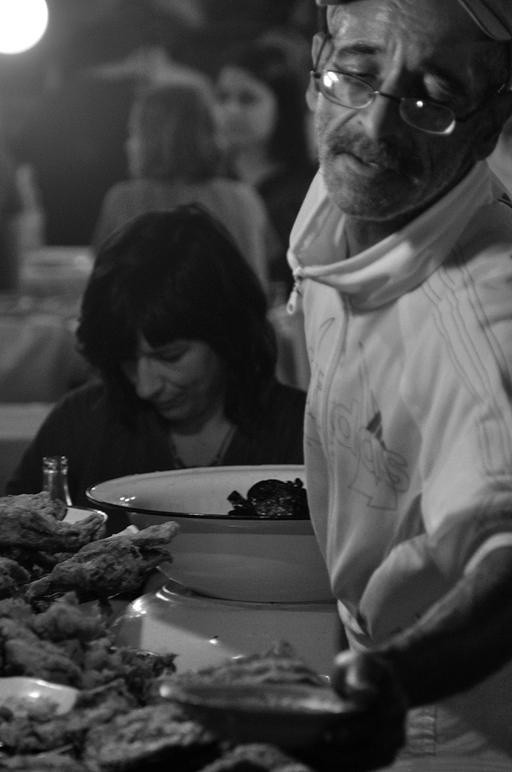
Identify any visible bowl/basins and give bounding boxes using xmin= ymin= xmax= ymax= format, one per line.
xmin=109 ymin=580 xmax=350 ymax=683
xmin=82 ymin=461 xmax=337 ymax=606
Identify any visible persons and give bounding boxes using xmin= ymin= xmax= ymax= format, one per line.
xmin=95 ymin=82 xmax=272 ymax=308
xmin=1 ymin=201 xmax=309 ymax=507
xmin=284 ymin=0 xmax=511 ymax=772
xmin=214 ymin=43 xmax=316 ymax=298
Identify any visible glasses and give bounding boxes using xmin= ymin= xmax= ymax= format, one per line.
xmin=307 ymin=36 xmax=506 ymax=138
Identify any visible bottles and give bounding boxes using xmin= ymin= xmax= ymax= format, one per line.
xmin=40 ymin=455 xmax=75 ymax=508
